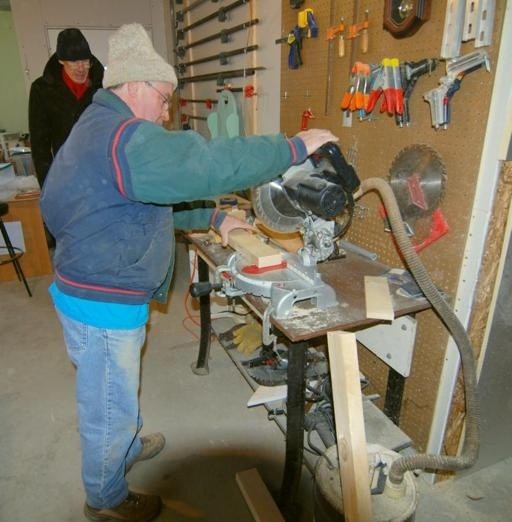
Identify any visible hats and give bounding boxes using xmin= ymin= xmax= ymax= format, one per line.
xmin=102 ymin=22 xmax=178 ymax=89
xmin=57 ymin=28 xmax=93 ymax=61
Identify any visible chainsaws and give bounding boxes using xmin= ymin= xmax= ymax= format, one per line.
xmin=188 ymin=140 xmax=362 ymax=345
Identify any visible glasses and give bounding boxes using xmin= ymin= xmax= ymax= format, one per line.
xmin=142 ymin=80 xmax=174 ymax=110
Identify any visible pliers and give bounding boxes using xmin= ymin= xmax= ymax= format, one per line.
xmin=340 ymin=57 xmax=404 ymax=114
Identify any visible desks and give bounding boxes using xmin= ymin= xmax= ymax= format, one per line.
xmin=179 ymin=208 xmax=451 ymax=521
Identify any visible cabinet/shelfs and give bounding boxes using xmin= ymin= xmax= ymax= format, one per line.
xmin=0 ymin=183 xmax=59 ymax=284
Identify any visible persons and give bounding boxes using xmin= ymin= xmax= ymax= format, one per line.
xmin=38 ymin=21 xmax=342 ymax=522
xmin=27 ymin=28 xmax=105 ymax=192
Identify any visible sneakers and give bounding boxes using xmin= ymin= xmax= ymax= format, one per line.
xmin=84 ymin=491 xmax=163 ymax=522
xmin=125 ymin=432 xmax=166 ymax=473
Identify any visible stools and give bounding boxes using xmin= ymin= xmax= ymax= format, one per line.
xmin=0 ymin=201 xmax=34 ymax=300
